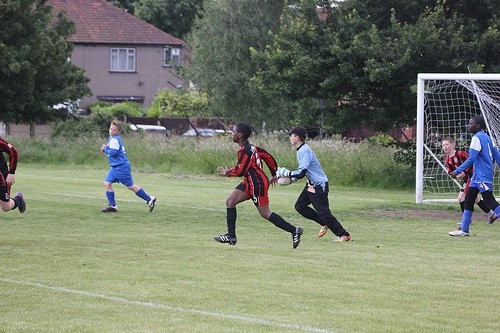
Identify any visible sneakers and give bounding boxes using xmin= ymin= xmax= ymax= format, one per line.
xmin=101 ymin=205 xmax=119 ymax=212
xmin=147 ymin=196 xmax=157 ymax=213
xmin=293 ymin=227 xmax=304 ymax=248
xmin=214 ymin=234 xmax=237 ymax=246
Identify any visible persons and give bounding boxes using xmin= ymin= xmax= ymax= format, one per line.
xmin=441 ymin=137 xmax=495 ymax=225
xmin=276 ymin=126 xmax=353 ymax=242
xmin=214 ymin=123 xmax=303 ymax=249
xmin=449 ymin=116 xmax=500 ymax=237
xmin=0 ymin=136 xmax=26 ymax=213
xmin=100 ymin=120 xmax=157 ymax=213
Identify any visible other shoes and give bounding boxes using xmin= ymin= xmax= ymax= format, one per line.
xmin=449 ymin=230 xmax=469 ymax=236
xmin=317 ymin=224 xmax=329 ymax=237
xmin=487 ymin=212 xmax=498 ymax=224
xmin=333 ymin=235 xmax=350 ymax=242
xmin=16 ymin=192 xmax=26 ymax=213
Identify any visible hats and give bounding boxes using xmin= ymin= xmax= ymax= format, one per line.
xmin=284 ymin=126 xmax=306 ymax=137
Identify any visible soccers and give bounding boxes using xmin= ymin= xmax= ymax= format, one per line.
xmin=278 ymin=177 xmax=291 ymax=186
